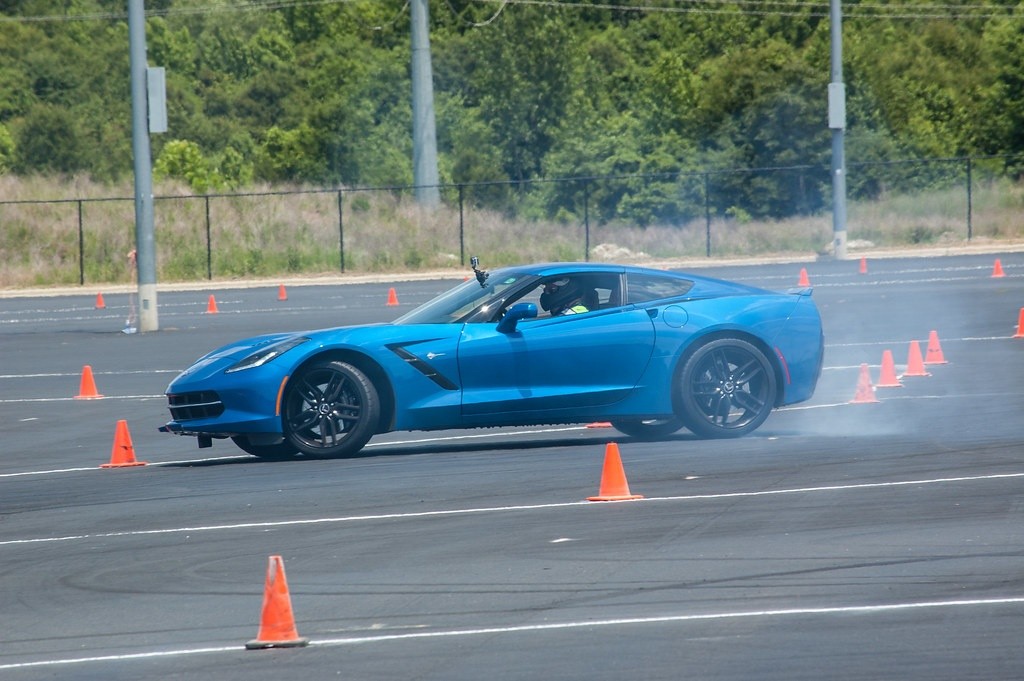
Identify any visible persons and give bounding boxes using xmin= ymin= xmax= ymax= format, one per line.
xmin=541 ymin=277 xmax=593 ymax=316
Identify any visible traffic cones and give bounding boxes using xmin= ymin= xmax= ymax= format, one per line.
xmin=796 ymin=267 xmax=811 ymax=289
xmin=990 ymin=259 xmax=1007 ymax=278
xmin=384 ymin=288 xmax=399 ymax=307
xmin=923 ymin=329 xmax=949 ymax=366
xmin=874 ymin=350 xmax=904 ymax=388
xmin=900 ymin=341 xmax=930 ymax=378
xmin=275 ymin=284 xmax=288 ymax=300
xmin=585 ymin=443 xmax=645 ymax=503
xmin=98 ymin=419 xmax=147 ymax=468
xmin=847 ymin=363 xmax=879 ymax=403
xmin=72 ymin=365 xmax=105 ymax=398
xmin=206 ymin=295 xmax=220 ymax=314
xmin=859 ymin=256 xmax=869 ymax=274
xmin=94 ymin=294 xmax=108 ymax=312
xmin=1010 ymin=307 xmax=1024 ymax=339
xmin=243 ymin=555 xmax=312 ymax=647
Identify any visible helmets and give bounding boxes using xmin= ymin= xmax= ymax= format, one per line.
xmin=540 ymin=277 xmax=578 ymax=311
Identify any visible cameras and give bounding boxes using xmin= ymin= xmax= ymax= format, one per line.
xmin=471 ymin=257 xmax=479 ymax=269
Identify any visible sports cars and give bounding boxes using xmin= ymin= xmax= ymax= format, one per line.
xmin=162 ymin=261 xmax=829 ymax=459
